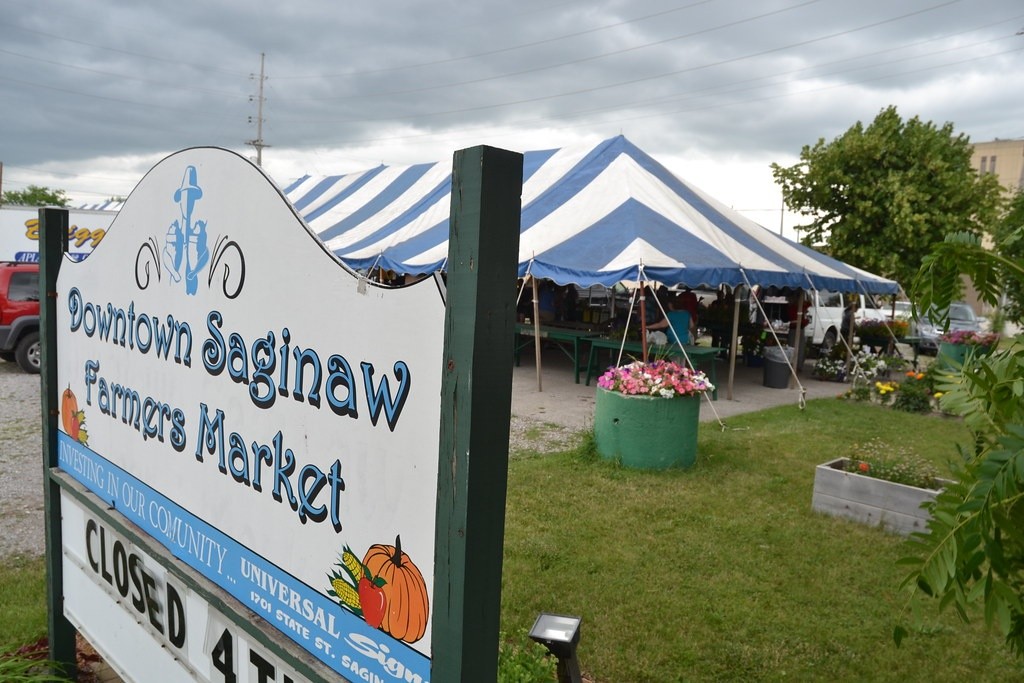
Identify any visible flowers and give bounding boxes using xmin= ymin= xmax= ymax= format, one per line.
xmin=812 ymin=357 xmax=848 ymax=376
xmin=941 ymin=329 xmax=999 ymax=345
xmin=837 ymin=438 xmax=936 ymax=490
xmin=855 ymin=319 xmax=906 ymax=335
xmin=875 ymin=351 xmax=909 ymax=371
xmin=594 ymin=340 xmax=717 ymax=401
xmin=860 ymin=368 xmax=955 ymax=400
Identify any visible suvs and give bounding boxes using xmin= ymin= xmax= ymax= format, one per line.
xmin=0 ymin=259 xmax=42 ymax=374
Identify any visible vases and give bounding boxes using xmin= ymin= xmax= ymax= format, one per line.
xmin=594 ymin=376 xmax=703 ymax=471
xmin=935 ymin=340 xmax=971 ymax=371
xmin=869 ymin=387 xmax=963 ymax=418
xmin=814 ymin=369 xmax=845 ymax=384
xmin=869 ymin=365 xmax=907 ymax=381
xmin=860 ymin=332 xmax=905 ymax=339
xmin=811 ymin=458 xmax=959 ymax=544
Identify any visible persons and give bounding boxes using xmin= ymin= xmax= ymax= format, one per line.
xmin=513 ymin=275 xmax=766 ymax=366
xmin=833 ymin=299 xmax=861 ymax=358
xmin=786 ymin=288 xmax=815 ymax=370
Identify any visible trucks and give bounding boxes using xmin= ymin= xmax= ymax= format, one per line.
xmin=1 ymin=205 xmax=120 ymax=265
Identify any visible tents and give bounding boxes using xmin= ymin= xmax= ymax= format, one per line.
xmin=261 ymin=130 xmax=906 ymax=397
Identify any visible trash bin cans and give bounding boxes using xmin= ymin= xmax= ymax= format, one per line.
xmin=762 ymin=344 xmax=796 ymax=389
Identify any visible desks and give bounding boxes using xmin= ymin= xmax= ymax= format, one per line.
xmin=515 ymin=323 xmax=604 ymax=384
xmin=580 ymin=337 xmax=726 ymax=401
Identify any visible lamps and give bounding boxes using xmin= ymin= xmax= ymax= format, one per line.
xmin=527 ymin=613 xmax=584 ymax=683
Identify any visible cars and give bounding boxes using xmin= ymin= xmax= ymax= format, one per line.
xmin=738 ymin=291 xmax=987 ymax=353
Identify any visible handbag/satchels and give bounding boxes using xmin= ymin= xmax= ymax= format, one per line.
xmin=695 ymin=335 xmax=712 ymax=348
